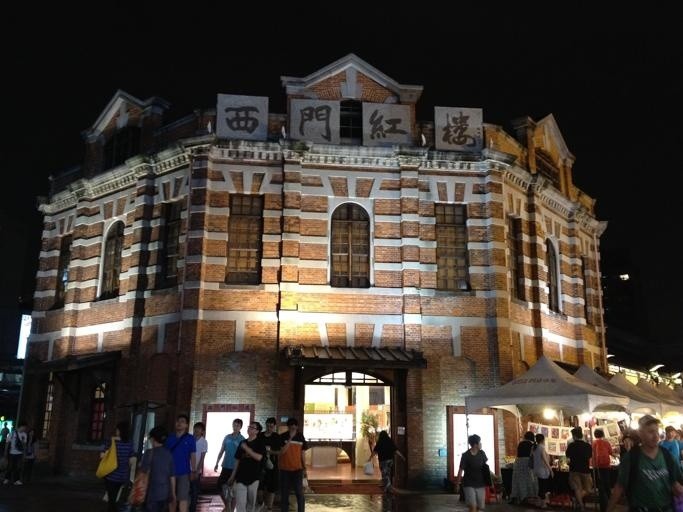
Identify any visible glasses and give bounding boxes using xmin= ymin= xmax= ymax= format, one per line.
xmin=248 ymin=426 xmax=257 ymax=430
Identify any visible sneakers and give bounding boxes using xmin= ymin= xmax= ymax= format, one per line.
xmin=13 ymin=479 xmax=24 ymax=486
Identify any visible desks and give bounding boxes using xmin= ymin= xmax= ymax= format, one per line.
xmin=501 ymin=466 xmax=618 ymax=511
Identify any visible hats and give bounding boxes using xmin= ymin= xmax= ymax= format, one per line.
xmin=639 ymin=415 xmax=660 ymax=425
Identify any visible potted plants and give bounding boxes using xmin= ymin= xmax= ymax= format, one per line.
xmin=70 ymin=436 xmax=104 ymax=472
xmin=360 ymin=409 xmax=380 ymax=468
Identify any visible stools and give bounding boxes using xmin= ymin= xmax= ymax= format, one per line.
xmin=486 ymin=480 xmax=498 ymax=505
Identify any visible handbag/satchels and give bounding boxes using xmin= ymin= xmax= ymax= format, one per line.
xmin=262 ymin=450 xmax=282 ymax=484
xmin=528 ymin=444 xmax=537 ymax=469
xmin=96 ymin=437 xmax=118 ymax=479
xmin=363 ymin=460 xmax=374 ymax=475
xmin=14 ymin=428 xmax=25 ymax=451
xmin=128 ymin=449 xmax=153 ymax=506
xmin=480 ymin=449 xmax=492 ymax=487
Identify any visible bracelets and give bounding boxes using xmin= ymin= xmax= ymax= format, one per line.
xmin=303 ymin=466 xmax=306 ymax=469
xmin=398 ymin=453 xmax=402 ymax=458
xmin=191 ymin=469 xmax=195 ymax=473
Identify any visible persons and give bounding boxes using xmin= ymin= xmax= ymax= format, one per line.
xmin=565 ymin=426 xmax=594 ymax=511
xmin=590 ymin=428 xmax=612 ymax=512
xmin=214 ymin=419 xmax=245 ymax=512
xmin=529 ymin=433 xmax=555 ymax=511
xmin=456 ymin=434 xmax=489 ymax=511
xmin=366 ymin=429 xmax=405 ymax=493
xmin=95 ymin=421 xmax=136 ymax=512
xmin=225 ymin=421 xmax=266 ymax=512
xmin=134 ymin=425 xmax=177 ymax=511
xmin=507 ymin=430 xmax=536 ymax=505
xmin=188 ymin=421 xmax=207 ymax=512
xmin=165 ymin=414 xmax=197 ymax=512
xmin=257 ymin=416 xmax=279 ymax=512
xmin=268 ymin=417 xmax=307 ymax=512
xmin=0 ymin=422 xmax=39 ymax=487
xmin=605 ymin=414 xmax=682 ymax=512
